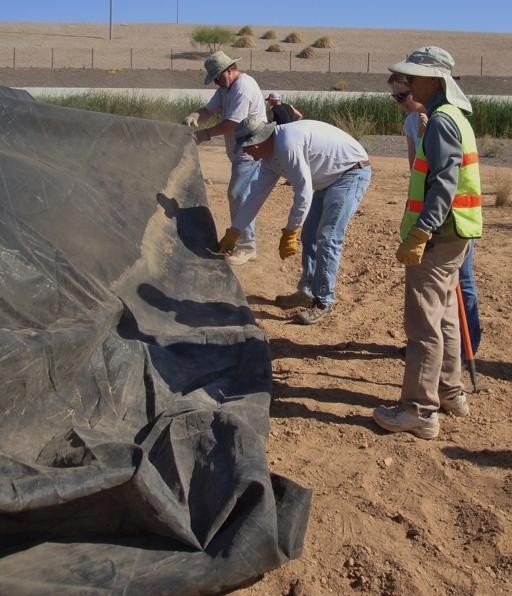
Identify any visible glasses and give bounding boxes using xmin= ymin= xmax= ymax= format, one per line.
xmin=391 ymin=90 xmax=410 ymax=101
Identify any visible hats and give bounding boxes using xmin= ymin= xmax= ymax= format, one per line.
xmin=265 ymin=91 xmax=281 ymax=101
xmin=203 ymin=51 xmax=243 ymax=85
xmin=388 ymin=46 xmax=472 ymax=116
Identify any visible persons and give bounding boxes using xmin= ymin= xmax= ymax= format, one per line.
xmin=374 ymin=46 xmax=484 ymax=439
xmin=182 ymin=51 xmax=269 ymax=264
xmin=387 ymin=72 xmax=480 ymax=373
xmin=214 ymin=116 xmax=373 ymax=325
xmin=266 ymin=92 xmax=302 ymax=124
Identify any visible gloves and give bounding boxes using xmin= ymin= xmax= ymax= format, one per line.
xmin=192 ymin=127 xmax=210 ymax=145
xmin=183 ymin=112 xmax=200 ymax=128
xmin=218 ymin=228 xmax=240 ymax=256
xmin=234 ymin=116 xmax=277 ymax=154
xmin=279 ymin=228 xmax=300 ymax=260
xmin=395 ymin=225 xmax=433 ymax=265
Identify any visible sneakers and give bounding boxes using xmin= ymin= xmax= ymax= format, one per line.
xmin=296 ymin=303 xmax=333 ymax=325
xmin=275 ymin=290 xmax=312 ymax=308
xmin=224 ymin=247 xmax=256 ymax=265
xmin=373 ymin=392 xmax=470 ymax=439
